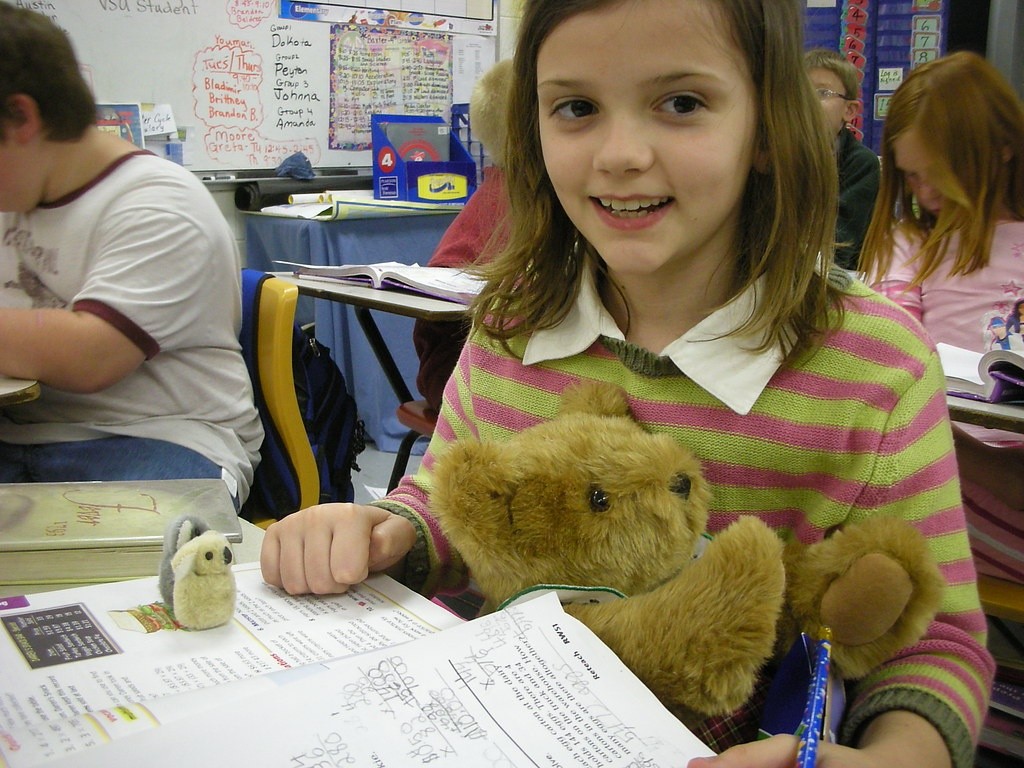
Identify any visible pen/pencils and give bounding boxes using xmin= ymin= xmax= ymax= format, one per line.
xmin=794 ymin=625 xmax=834 ymax=767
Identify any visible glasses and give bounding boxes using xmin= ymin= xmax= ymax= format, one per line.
xmin=815 ymin=88 xmax=855 ymax=101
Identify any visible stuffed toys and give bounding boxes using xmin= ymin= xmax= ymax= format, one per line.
xmin=428 ymin=379 xmax=946 ymax=754
xmin=159 ymin=514 xmax=236 ymax=629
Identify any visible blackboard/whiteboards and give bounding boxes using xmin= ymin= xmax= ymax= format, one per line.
xmin=0 ymin=0 xmax=501 ymax=193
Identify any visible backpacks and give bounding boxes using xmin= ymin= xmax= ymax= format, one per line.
xmin=237 ymin=265 xmax=368 ymax=517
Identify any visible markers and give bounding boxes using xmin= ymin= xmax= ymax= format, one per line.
xmin=201 ymin=174 xmax=237 ymax=179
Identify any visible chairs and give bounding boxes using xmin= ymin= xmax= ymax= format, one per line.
xmin=236 ymin=264 xmax=324 ymax=535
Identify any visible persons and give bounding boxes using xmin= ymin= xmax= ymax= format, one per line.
xmin=803 ymin=49 xmax=880 ymax=270
xmin=413 ymin=58 xmax=513 ymax=421
xmin=0 ymin=0 xmax=264 ymax=513
xmin=259 ymin=1 xmax=997 ymax=768
xmin=857 ymin=51 xmax=1024 ymax=586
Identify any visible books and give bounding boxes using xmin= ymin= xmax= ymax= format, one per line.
xmin=978 ymin=674 xmax=1024 ymax=759
xmin=936 ymin=341 xmax=1024 ymax=404
xmin=272 ymin=260 xmax=489 ymax=305
xmin=0 ymin=479 xmax=244 ymax=586
xmin=0 ymin=560 xmax=717 ymax=768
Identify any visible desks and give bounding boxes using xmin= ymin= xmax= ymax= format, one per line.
xmin=268 ymin=266 xmax=472 ymax=497
xmin=243 ymin=204 xmax=467 ymax=458
